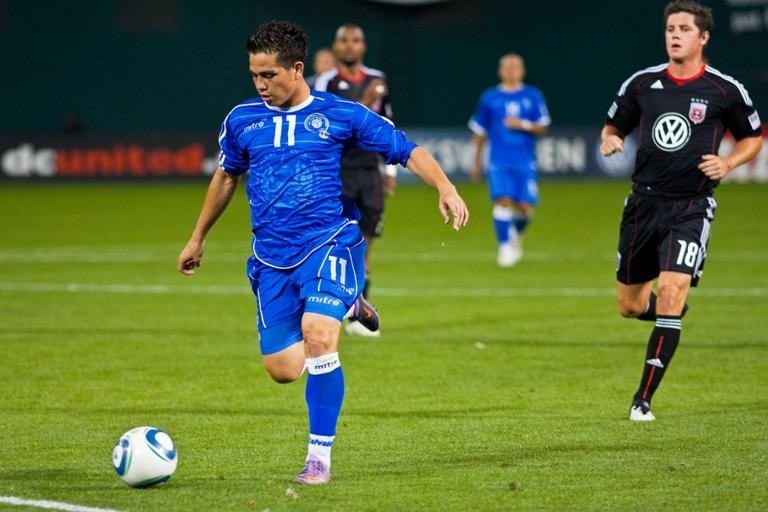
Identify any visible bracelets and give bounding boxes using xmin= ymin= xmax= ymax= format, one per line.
xmin=521 ymin=119 xmax=532 ymax=131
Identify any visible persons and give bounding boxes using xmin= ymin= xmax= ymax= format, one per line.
xmin=305 ymin=47 xmax=336 ymax=91
xmin=312 ymin=24 xmax=390 ymax=335
xmin=177 ymin=20 xmax=469 ymax=484
xmin=467 ymin=53 xmax=552 ymax=268
xmin=384 ymin=95 xmax=396 ymax=195
xmin=600 ymin=1 xmax=763 ymax=423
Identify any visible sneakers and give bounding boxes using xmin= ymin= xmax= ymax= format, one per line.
xmin=292 ymin=454 xmax=330 ymax=487
xmin=497 ymin=243 xmax=514 ymax=268
xmin=509 ymin=226 xmax=521 ymax=262
xmin=345 ymin=320 xmax=380 ymax=337
xmin=348 ymin=292 xmax=379 ymax=332
xmin=628 ymin=391 xmax=655 ymax=423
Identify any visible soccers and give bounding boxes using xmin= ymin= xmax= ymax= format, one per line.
xmin=110 ymin=425 xmax=179 ymax=488
